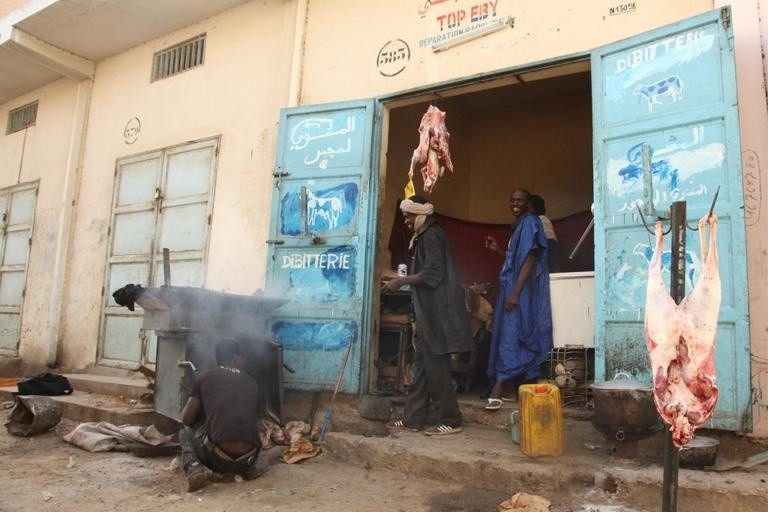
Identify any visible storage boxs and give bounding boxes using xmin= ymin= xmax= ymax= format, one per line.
xmin=548 ymin=270 xmax=596 ymax=349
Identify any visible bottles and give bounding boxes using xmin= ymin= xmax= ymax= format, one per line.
xmin=397 ymin=265 xmax=408 ymax=291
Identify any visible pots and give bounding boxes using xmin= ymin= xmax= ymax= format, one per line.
xmin=587 ymin=373 xmax=659 ymax=434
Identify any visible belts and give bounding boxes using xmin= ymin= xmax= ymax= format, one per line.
xmin=203 ymin=436 xmax=257 ymax=462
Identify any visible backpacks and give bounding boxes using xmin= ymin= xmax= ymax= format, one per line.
xmin=13 ymin=372 xmax=73 ymax=395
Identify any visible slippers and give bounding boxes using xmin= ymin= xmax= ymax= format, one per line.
xmin=501 ymin=397 xmax=518 ymax=401
xmin=485 ymin=398 xmax=504 ymax=410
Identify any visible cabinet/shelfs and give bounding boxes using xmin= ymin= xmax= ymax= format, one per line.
xmin=375 ymin=286 xmax=480 ymax=395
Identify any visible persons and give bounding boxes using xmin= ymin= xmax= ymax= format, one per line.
xmin=174 ymin=338 xmax=266 ymax=491
xmin=484 ymin=187 xmax=555 ymax=411
xmin=528 ymin=193 xmax=566 ymax=274
xmin=378 ymin=196 xmax=476 ymax=434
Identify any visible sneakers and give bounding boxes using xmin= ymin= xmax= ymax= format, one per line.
xmin=386 ymin=415 xmax=419 ymax=432
xmin=187 ymin=465 xmax=213 ymax=488
xmin=423 ymin=424 xmax=462 ymax=435
xmin=245 ymin=463 xmax=264 ymax=480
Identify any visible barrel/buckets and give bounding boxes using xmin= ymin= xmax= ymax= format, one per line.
xmin=518 ymin=384 xmax=564 ymax=458
